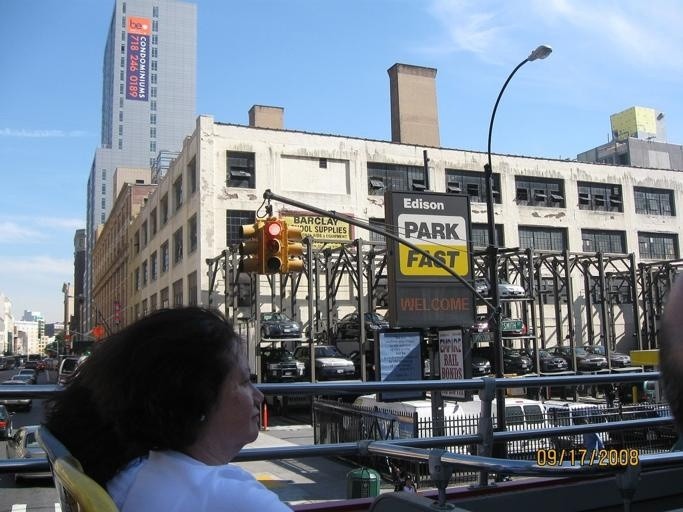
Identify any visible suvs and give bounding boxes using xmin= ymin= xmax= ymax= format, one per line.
xmin=293 ymin=344 xmax=355 ymax=380
xmin=0 ymin=379 xmax=33 ymax=412
xmin=540 ymin=397 xmax=612 ymax=445
xmin=260 ymin=346 xmax=305 ymax=382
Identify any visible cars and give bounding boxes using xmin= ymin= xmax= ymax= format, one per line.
xmin=610 ymin=404 xmax=676 ymax=444
xmin=4 ymin=423 xmax=47 ymax=461
xmin=0 ymin=404 xmax=17 ymax=439
xmin=349 ymin=346 xmax=374 ymax=378
xmin=0 ymin=352 xmax=77 ymax=386
xmin=259 ymin=311 xmax=303 ymax=339
xmin=473 ymin=277 xmax=631 ymax=375
xmin=334 ymin=310 xmax=390 ymax=339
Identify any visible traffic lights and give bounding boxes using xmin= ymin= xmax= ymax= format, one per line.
xmin=284 ymin=221 xmax=305 ymax=274
xmin=260 ymin=220 xmax=289 ymax=275
xmin=238 ymin=223 xmax=264 ymax=275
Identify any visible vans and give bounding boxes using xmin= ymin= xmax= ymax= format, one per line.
xmin=490 ymin=397 xmax=552 ymax=454
xmin=641 ymin=380 xmax=656 ymax=401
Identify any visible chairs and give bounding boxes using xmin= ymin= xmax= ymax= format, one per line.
xmin=34 ymin=426 xmax=121 ymax=512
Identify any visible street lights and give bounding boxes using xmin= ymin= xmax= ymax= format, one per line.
xmin=483 ymin=43 xmax=553 ymax=483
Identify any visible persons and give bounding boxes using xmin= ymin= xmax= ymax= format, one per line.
xmin=401 ymin=478 xmax=416 ymax=493
xmin=393 ymin=472 xmax=415 ymax=492
xmin=38 ymin=303 xmax=294 ymax=512
xmin=657 ymin=267 xmax=682 ymax=436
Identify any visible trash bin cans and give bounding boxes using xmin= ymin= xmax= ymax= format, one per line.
xmin=346 ymin=467 xmax=380 ymax=499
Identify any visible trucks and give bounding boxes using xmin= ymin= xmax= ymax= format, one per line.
xmin=339 ymin=393 xmax=470 ymax=469
